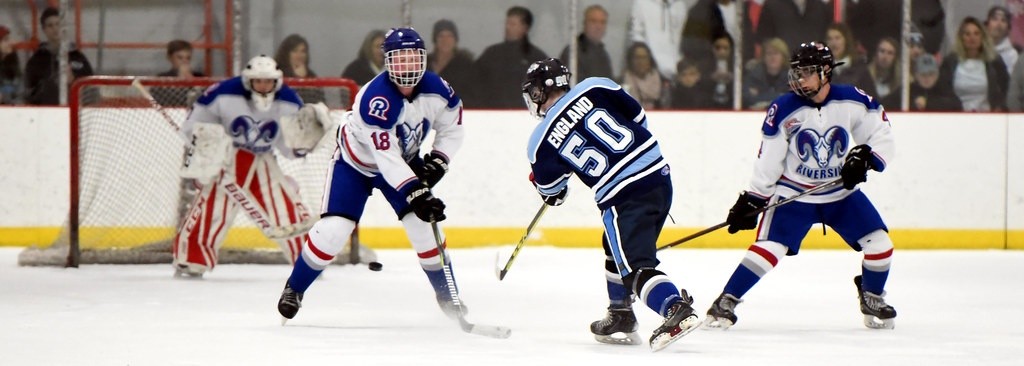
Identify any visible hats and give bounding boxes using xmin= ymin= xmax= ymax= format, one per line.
xmin=916 ymin=54 xmax=937 ymax=72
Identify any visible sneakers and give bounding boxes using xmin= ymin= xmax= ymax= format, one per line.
xmin=855 ymin=274 xmax=896 ymax=329
xmin=708 ymin=292 xmax=743 ymax=330
xmin=440 ymin=298 xmax=468 ymax=323
xmin=277 ymin=276 xmax=304 ymax=328
xmin=649 ymin=288 xmax=702 ymax=353
xmin=590 ymin=293 xmax=640 ymax=345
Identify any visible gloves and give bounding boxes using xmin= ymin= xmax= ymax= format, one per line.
xmin=840 ymin=143 xmax=872 ymax=190
xmin=407 ymin=185 xmax=445 ymax=223
xmin=542 ymin=187 xmax=568 ymax=206
xmin=727 ymin=189 xmax=771 ymax=235
xmin=417 ymin=154 xmax=448 ymax=188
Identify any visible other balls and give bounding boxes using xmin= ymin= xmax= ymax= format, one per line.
xmin=369 ymin=262 xmax=382 ymax=271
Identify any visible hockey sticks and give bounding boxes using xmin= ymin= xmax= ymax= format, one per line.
xmin=499 ymin=203 xmax=547 ymax=281
xmin=429 ymin=213 xmax=511 ymax=340
xmin=131 ymin=79 xmax=329 ymax=242
xmin=656 ymin=178 xmax=844 ymax=252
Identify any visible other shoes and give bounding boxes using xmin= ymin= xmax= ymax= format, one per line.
xmin=176 ymin=263 xmax=203 ymax=280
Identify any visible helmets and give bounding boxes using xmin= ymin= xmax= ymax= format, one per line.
xmin=521 ymin=57 xmax=571 ymax=120
xmin=788 ymin=41 xmax=833 ymax=99
xmin=380 ymin=26 xmax=427 ymax=89
xmin=240 ymin=54 xmax=284 ymax=113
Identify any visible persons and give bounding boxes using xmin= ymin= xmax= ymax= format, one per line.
xmin=468 ymin=6 xmax=551 ymax=110
xmin=340 ymin=30 xmax=387 ymax=109
xmin=426 ymin=19 xmax=470 ymax=110
xmin=701 ymin=41 xmax=897 ymax=332
xmin=277 ymin=28 xmax=467 ymax=326
xmin=665 ymin=58 xmax=715 ymax=111
xmin=617 ymin=42 xmax=675 ymax=111
xmin=0 ymin=25 xmax=22 ymax=106
xmin=149 ymin=40 xmax=210 ymax=108
xmin=560 ymin=5 xmax=618 ymax=85
xmin=172 ymin=54 xmax=332 ymax=278
xmin=25 ymin=7 xmax=99 ymax=106
xmin=908 ymin=6 xmax=1024 ymax=112
xmin=521 ymin=58 xmax=702 ymax=351
xmin=274 ymin=33 xmax=329 ymax=107
xmin=681 ymin=0 xmax=903 ymax=112
